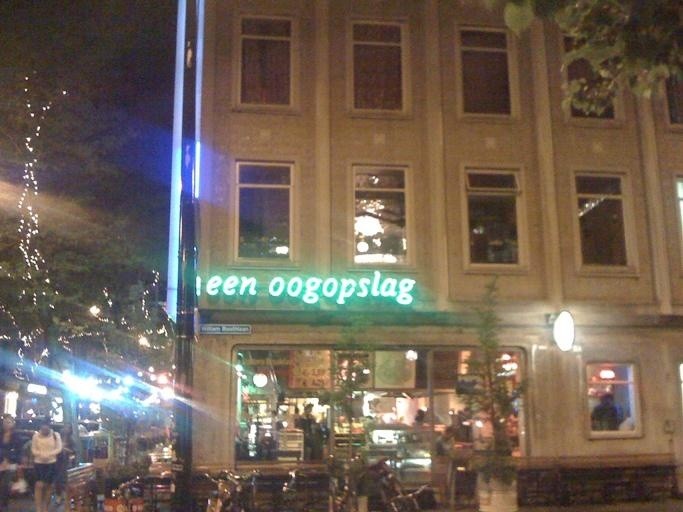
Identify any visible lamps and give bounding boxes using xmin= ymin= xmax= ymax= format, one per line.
xmin=404 ymin=350 xmax=417 ymax=361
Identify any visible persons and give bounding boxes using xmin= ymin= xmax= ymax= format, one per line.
xmin=31 ymin=422 xmax=63 ymax=512
xmin=0 ymin=428 xmax=21 ymax=510
xmin=55 ymin=423 xmax=75 ymax=505
xmin=591 ymin=392 xmax=623 ymax=430
xmin=300 ymin=404 xmax=314 ymax=420
xmin=436 ymin=428 xmax=456 ymax=452
xmin=415 ymin=409 xmax=424 ymax=420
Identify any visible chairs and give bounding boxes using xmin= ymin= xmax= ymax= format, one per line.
xmin=107 ymin=466 xmax=433 ymax=512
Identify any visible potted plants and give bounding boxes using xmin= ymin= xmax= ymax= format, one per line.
xmin=433 ymin=271 xmax=531 ymax=512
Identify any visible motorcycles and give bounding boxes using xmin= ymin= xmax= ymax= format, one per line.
xmin=193 ymin=453 xmax=426 ymax=511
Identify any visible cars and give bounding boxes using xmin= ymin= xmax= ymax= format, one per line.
xmin=6 ymin=417 xmax=98 ymax=454
